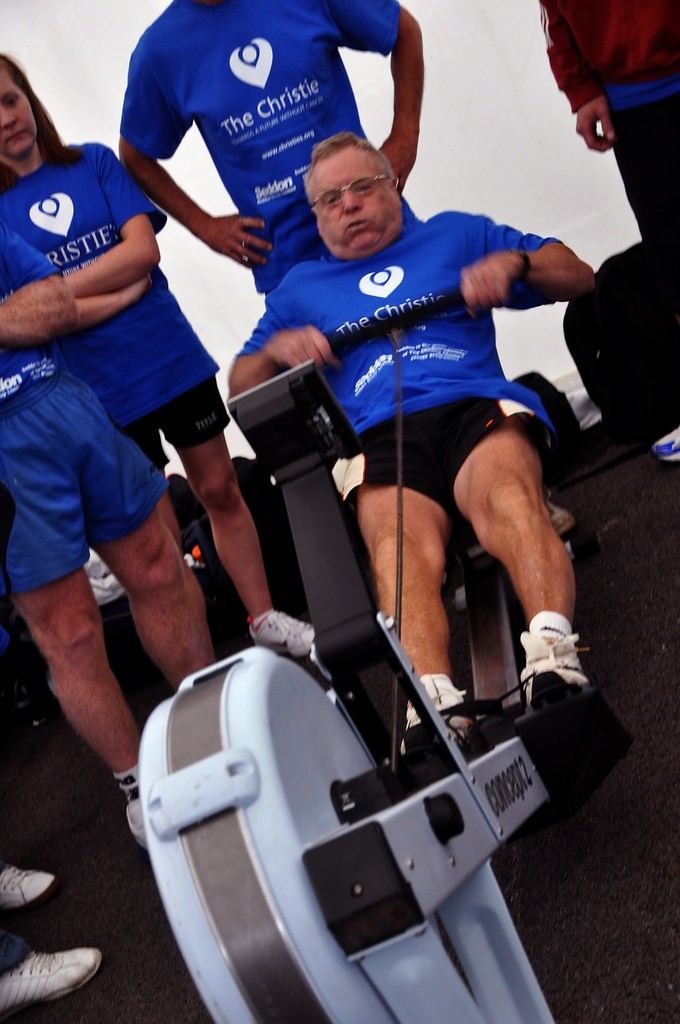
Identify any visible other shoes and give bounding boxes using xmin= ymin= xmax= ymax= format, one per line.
xmin=545 ymin=499 xmax=575 ymax=534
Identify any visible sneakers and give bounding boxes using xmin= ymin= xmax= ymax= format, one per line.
xmin=651 ymin=425 xmax=680 ymax=461
xmin=0 ymin=864 xmax=59 ymax=912
xmin=0 ymin=947 xmax=102 ymax=1021
xmin=519 ymin=631 xmax=589 ymax=710
xmin=399 ymin=673 xmax=472 ymax=756
xmin=247 ymin=610 xmax=315 ymax=656
xmin=126 ymin=805 xmax=148 ymax=851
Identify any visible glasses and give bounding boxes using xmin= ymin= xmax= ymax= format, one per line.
xmin=313 ymin=174 xmax=391 ymax=207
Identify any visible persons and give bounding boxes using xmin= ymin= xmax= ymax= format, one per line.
xmin=540 ymin=0 xmax=680 ymax=460
xmin=0 ymin=54 xmax=317 ymax=846
xmin=228 ymin=135 xmax=593 ymax=748
xmin=117 ymin=0 xmax=576 ymax=609
xmin=0 ymin=864 xmax=102 ymax=1020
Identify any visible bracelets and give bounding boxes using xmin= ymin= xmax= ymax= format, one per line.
xmin=512 ymin=250 xmax=530 ymax=283
